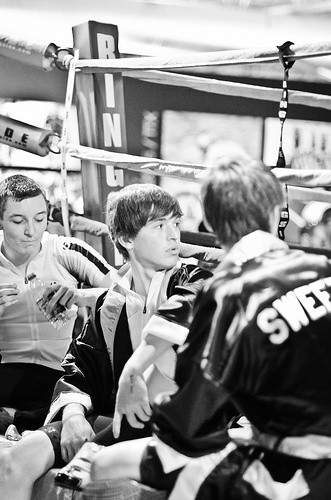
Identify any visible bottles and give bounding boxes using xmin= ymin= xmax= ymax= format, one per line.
xmin=25 ymin=273 xmax=72 ymax=330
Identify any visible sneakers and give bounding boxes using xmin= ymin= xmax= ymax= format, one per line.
xmin=54 ymin=441 xmax=108 ymax=491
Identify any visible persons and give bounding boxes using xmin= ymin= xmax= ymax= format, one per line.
xmin=1 ymin=176 xmax=122 ymax=436
xmin=1 ymin=182 xmax=215 ymax=500
xmin=89 ymin=159 xmax=331 ymax=500
